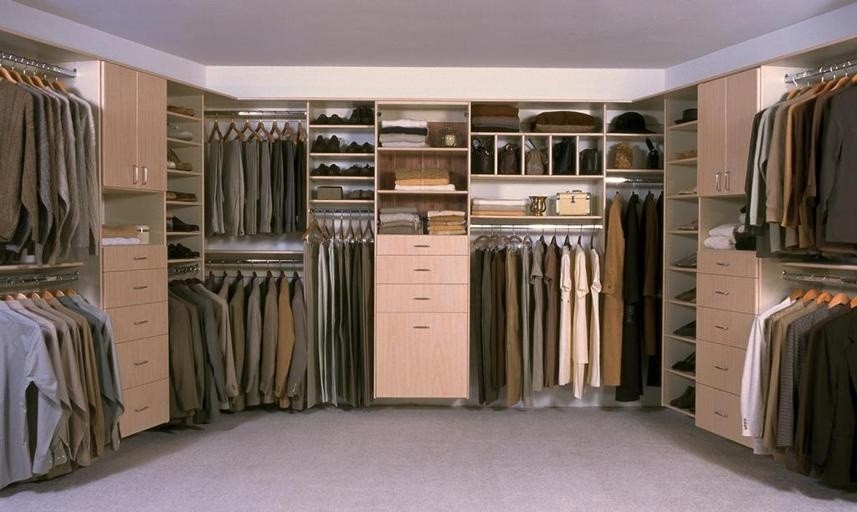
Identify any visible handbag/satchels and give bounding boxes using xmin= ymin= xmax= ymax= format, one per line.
xmin=498 ymin=143 xmax=521 ymax=175
xmin=426 ymin=120 xmax=468 ymax=148
xmin=610 ymin=142 xmax=633 ymax=169
xmin=554 ymin=136 xmax=575 ymax=175
xmin=644 ymin=139 xmax=659 ymax=169
xmin=579 ymin=148 xmax=600 ymax=175
xmin=471 ymin=137 xmax=494 ymax=174
xmin=525 ymin=138 xmax=546 ymax=175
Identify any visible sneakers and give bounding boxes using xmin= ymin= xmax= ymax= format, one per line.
xmin=167 ymin=101 xmax=201 ymax=260
xmin=310 ymin=103 xmax=376 ymax=200
xmin=667 ymin=148 xmax=698 ymax=413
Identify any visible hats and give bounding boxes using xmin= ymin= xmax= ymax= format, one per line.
xmin=614 ymin=112 xmax=655 ymax=134
xmin=674 ymin=109 xmax=697 ymax=124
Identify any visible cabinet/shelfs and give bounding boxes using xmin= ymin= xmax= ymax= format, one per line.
xmin=1 ymin=28 xmax=857 ymax=453
